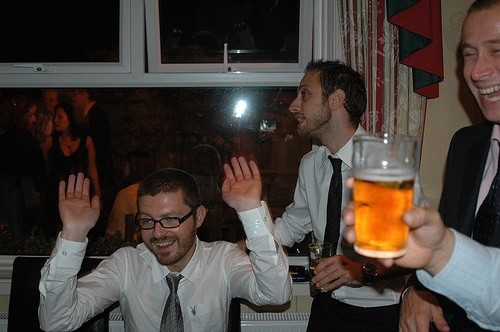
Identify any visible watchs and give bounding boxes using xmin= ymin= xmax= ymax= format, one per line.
xmin=360 ymin=260 xmax=378 ymax=285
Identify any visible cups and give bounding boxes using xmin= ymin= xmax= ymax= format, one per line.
xmin=351 ymin=133 xmax=416 ymax=259
xmin=307 ymin=242 xmax=332 ymax=299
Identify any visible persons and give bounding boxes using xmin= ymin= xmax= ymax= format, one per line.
xmin=11 ymin=88 xmax=286 ymax=249
xmin=398 ymin=0 xmax=500 ymax=332
xmin=341 ymin=176 xmax=500 ymax=331
xmin=233 ymin=59 xmax=430 ymax=332
xmin=38 ymin=157 xmax=293 ymax=332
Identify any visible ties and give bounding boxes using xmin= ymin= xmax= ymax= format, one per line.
xmin=323 ymin=156 xmax=342 ymax=259
xmin=160 ymin=274 xmax=186 ymax=332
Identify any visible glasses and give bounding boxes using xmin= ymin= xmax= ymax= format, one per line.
xmin=133 ymin=208 xmax=195 ymax=230
